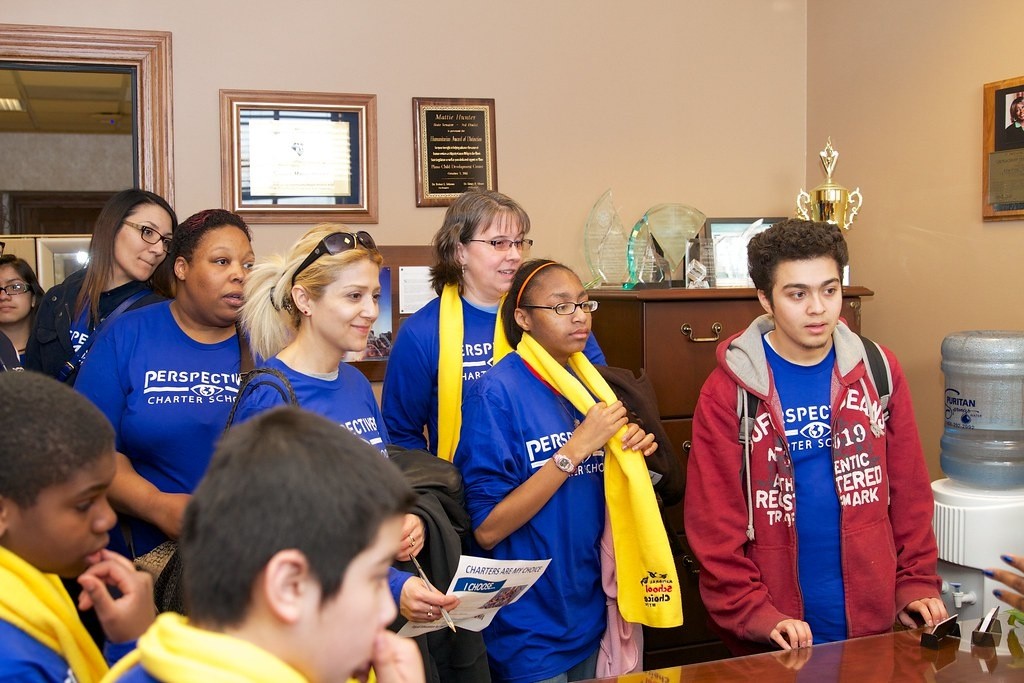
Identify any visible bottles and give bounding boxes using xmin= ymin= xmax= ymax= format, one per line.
xmin=940 ymin=330 xmax=1024 ymax=490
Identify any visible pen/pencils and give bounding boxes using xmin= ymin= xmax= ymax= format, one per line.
xmin=407 ymin=552 xmax=456 ymax=634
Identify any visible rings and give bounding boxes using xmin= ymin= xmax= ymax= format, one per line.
xmin=409 ymin=535 xmax=416 ymax=546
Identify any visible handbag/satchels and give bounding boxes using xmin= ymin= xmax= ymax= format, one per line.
xmin=132 ymin=537 xmax=187 ymax=616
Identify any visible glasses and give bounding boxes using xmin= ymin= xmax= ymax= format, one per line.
xmin=121 ymin=220 xmax=173 ymax=254
xmin=519 ymin=301 xmax=600 ymax=314
xmin=469 ymin=239 xmax=533 ymax=249
xmin=0 ymin=284 xmax=30 ymax=295
xmin=292 ymin=230 xmax=375 ymax=287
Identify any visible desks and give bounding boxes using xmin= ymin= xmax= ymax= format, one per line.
xmin=577 ymin=607 xmax=1024 ymax=683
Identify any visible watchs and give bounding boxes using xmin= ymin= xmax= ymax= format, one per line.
xmin=552 ymin=452 xmax=577 ymax=473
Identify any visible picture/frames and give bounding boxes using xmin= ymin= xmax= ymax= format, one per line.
xmin=705 ymin=216 xmax=789 ymax=287
xmin=981 ymin=76 xmax=1024 ymax=223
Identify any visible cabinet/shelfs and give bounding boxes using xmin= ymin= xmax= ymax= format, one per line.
xmin=585 ymin=285 xmax=875 ymax=672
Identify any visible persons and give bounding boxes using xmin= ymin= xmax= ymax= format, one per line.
xmin=453 ymin=259 xmax=657 ymax=683
xmin=98 ymin=405 xmax=425 ymax=683
xmin=382 ymin=189 xmax=607 ymax=463
xmin=982 ymin=554 xmax=1024 ymax=611
xmin=684 ymin=218 xmax=949 ymax=650
xmin=0 ymin=188 xmax=459 ymax=654
xmin=0 ymin=371 xmax=157 ymax=683
xmin=1005 ymin=97 xmax=1024 ymax=150
